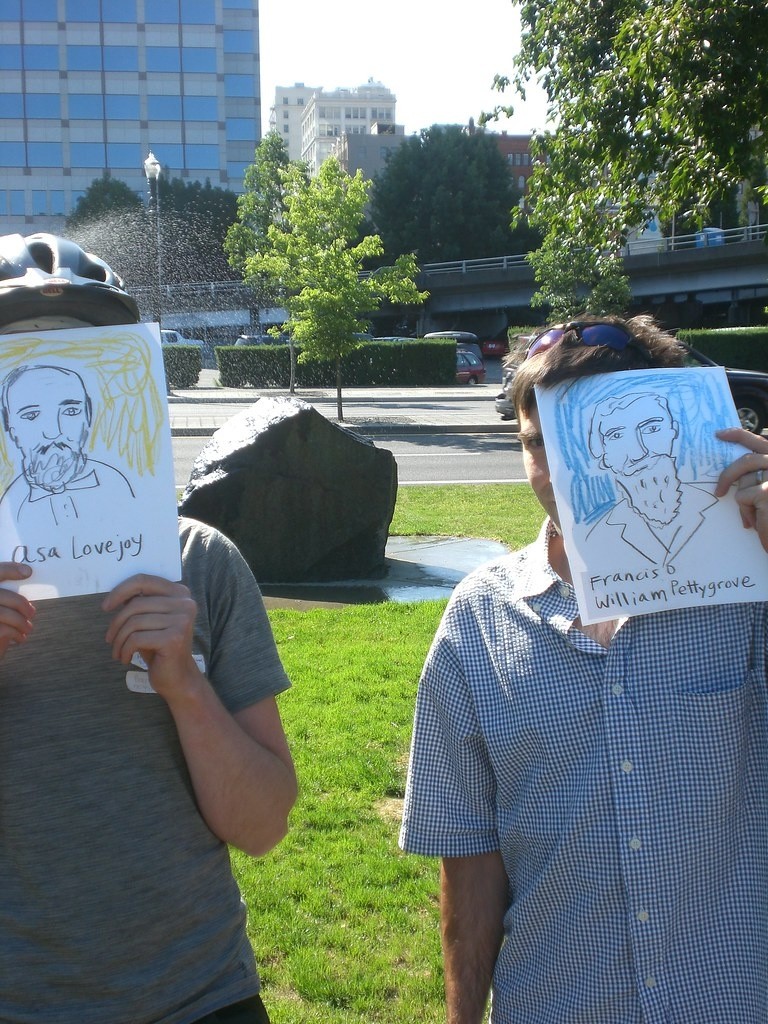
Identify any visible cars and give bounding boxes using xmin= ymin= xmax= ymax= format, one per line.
xmin=158 ymin=330 xmax=290 ymax=359
xmin=354 ymin=320 xmax=534 ymax=384
xmin=672 ymin=331 xmax=768 ymax=436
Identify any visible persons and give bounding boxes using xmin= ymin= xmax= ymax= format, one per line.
xmin=0 ymin=233 xmax=299 ymax=1024
xmin=399 ymin=315 xmax=768 ymax=1024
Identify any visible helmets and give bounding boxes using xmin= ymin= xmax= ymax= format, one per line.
xmin=0 ymin=233 xmax=140 ymax=322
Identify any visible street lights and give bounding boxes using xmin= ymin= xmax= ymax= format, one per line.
xmin=143 ymin=149 xmax=161 ymax=324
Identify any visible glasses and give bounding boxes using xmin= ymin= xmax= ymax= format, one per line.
xmin=524 ymin=321 xmax=662 ymax=367
